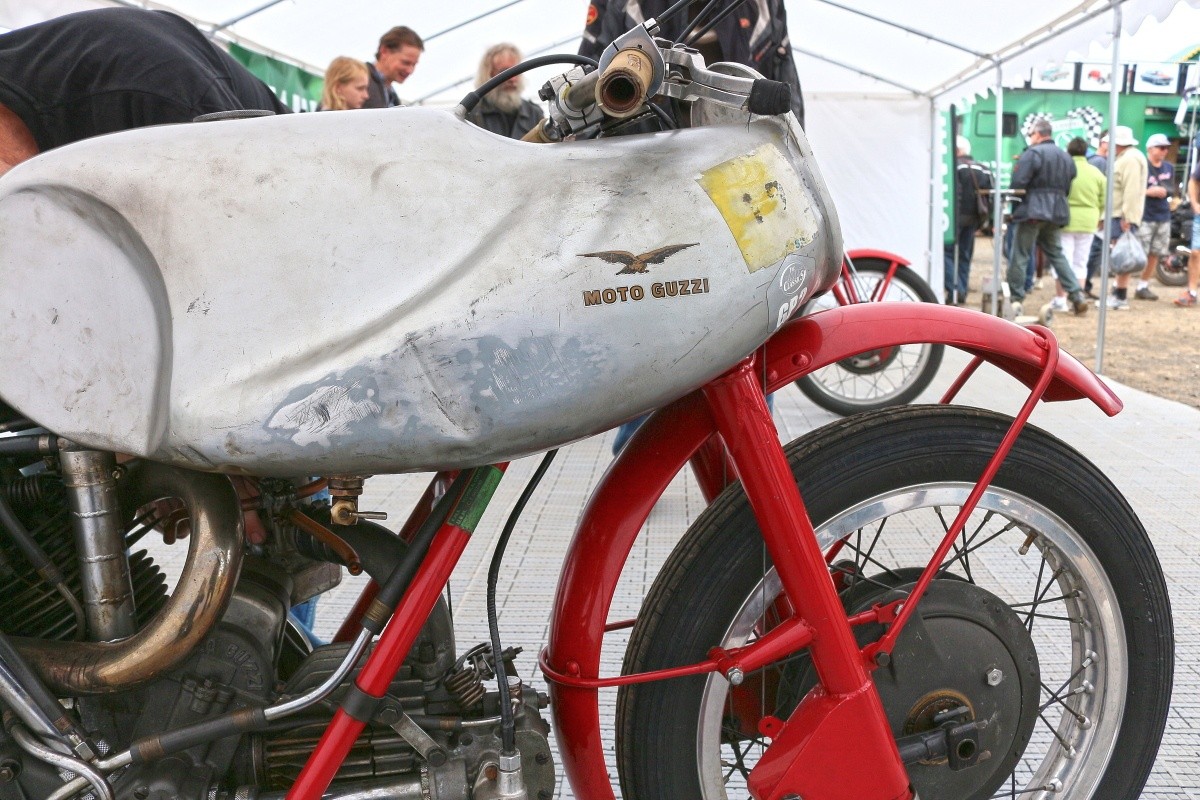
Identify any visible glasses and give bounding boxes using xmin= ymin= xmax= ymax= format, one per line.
xmin=1160 ymin=147 xmax=1170 ymax=150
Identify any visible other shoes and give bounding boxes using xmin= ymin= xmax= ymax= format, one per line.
xmin=1092 ymin=293 xmax=1131 ymax=310
xmin=1041 ymin=297 xmax=1070 ymax=314
xmin=1134 ymin=288 xmax=1158 ymax=302
xmin=1174 ymin=291 xmax=1198 ymax=306
xmin=1010 ymin=302 xmax=1024 ymax=316
xmin=945 ymin=288 xmax=955 ymax=305
xmin=956 ymin=291 xmax=967 ymax=303
xmin=1074 ymin=297 xmax=1092 ymax=314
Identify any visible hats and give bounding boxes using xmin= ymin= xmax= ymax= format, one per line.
xmin=1101 ymin=126 xmax=1141 ymax=146
xmin=1146 ymin=133 xmax=1173 ymax=148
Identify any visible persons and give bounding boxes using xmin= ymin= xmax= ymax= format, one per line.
xmin=320 ymin=57 xmax=370 ymax=112
xmin=0 ymin=7 xmax=337 ymax=644
xmin=462 ymin=44 xmax=544 ymax=140
xmin=571 ymin=0 xmax=806 ymax=460
xmin=945 ymin=111 xmax=1200 ymax=318
xmin=353 ymin=26 xmax=425 ymax=108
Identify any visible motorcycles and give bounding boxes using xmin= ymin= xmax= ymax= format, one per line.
xmin=0 ymin=0 xmax=1178 ymax=800
xmin=1154 ymin=182 xmax=1195 ymax=287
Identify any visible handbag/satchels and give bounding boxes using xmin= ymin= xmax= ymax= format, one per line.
xmin=976 ymin=189 xmax=990 ymax=226
xmin=1110 ymin=230 xmax=1148 ymax=273
